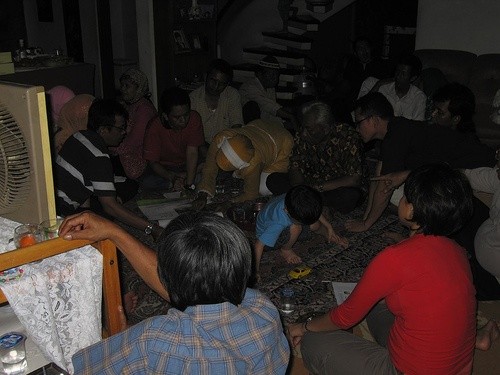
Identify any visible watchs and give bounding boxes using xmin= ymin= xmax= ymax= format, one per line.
xmin=144 ymin=224 xmax=154 ymax=235
xmin=183 ymin=183 xmax=196 ymax=191
xmin=318 ymin=185 xmax=326 ymax=193
xmin=304 ymin=317 xmax=318 ymax=332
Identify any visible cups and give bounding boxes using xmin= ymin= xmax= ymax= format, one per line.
xmin=0 ymin=332 xmax=28 ymax=375
xmin=14 ymin=224 xmax=42 ymax=249
xmin=39 ymin=219 xmax=64 ymax=241
xmin=279 ymin=290 xmax=295 ymax=313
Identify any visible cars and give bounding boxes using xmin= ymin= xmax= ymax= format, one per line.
xmin=287 ymin=266 xmax=311 ymax=280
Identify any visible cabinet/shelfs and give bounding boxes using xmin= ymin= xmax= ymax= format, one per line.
xmin=1 ymin=216 xmax=128 ymax=375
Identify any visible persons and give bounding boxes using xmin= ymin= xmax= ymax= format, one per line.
xmin=453 ymin=147 xmax=500 ymax=287
xmin=238 ymin=56 xmax=295 ymax=126
xmin=378 ymin=54 xmax=436 ymax=123
xmin=52 ymin=96 xmax=167 ymax=239
xmin=57 ymin=209 xmax=291 ymax=375
xmin=141 ymin=87 xmax=205 ymax=200
xmin=252 ymin=183 xmax=350 ymax=287
xmin=265 ymin=102 xmax=372 ymax=222
xmin=328 ymin=36 xmax=393 ymax=106
xmin=188 ymin=58 xmax=244 ymax=191
xmin=174 ymin=32 xmax=187 ymax=49
xmin=342 ymin=92 xmax=500 ymax=233
xmin=44 ymin=84 xmax=76 ymax=129
xmin=284 ymin=163 xmax=478 ymax=375
xmin=430 ymin=81 xmax=477 ymax=134
xmin=49 ymin=94 xmax=96 ymax=159
xmin=112 ymin=69 xmax=162 ymax=178
xmin=191 ymin=117 xmax=295 ymax=210
